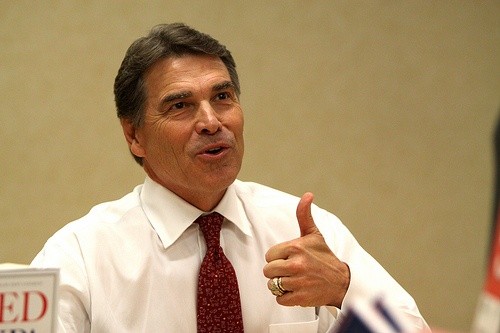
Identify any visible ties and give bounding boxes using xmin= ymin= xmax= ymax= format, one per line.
xmin=193 ymin=212 xmax=245 ymax=333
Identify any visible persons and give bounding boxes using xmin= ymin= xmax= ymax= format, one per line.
xmin=28 ymin=18 xmax=435 ymax=332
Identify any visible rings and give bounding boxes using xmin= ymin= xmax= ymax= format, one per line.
xmin=271 ymin=276 xmax=285 ymax=295
xmin=270 ymin=284 xmax=284 ymax=297
